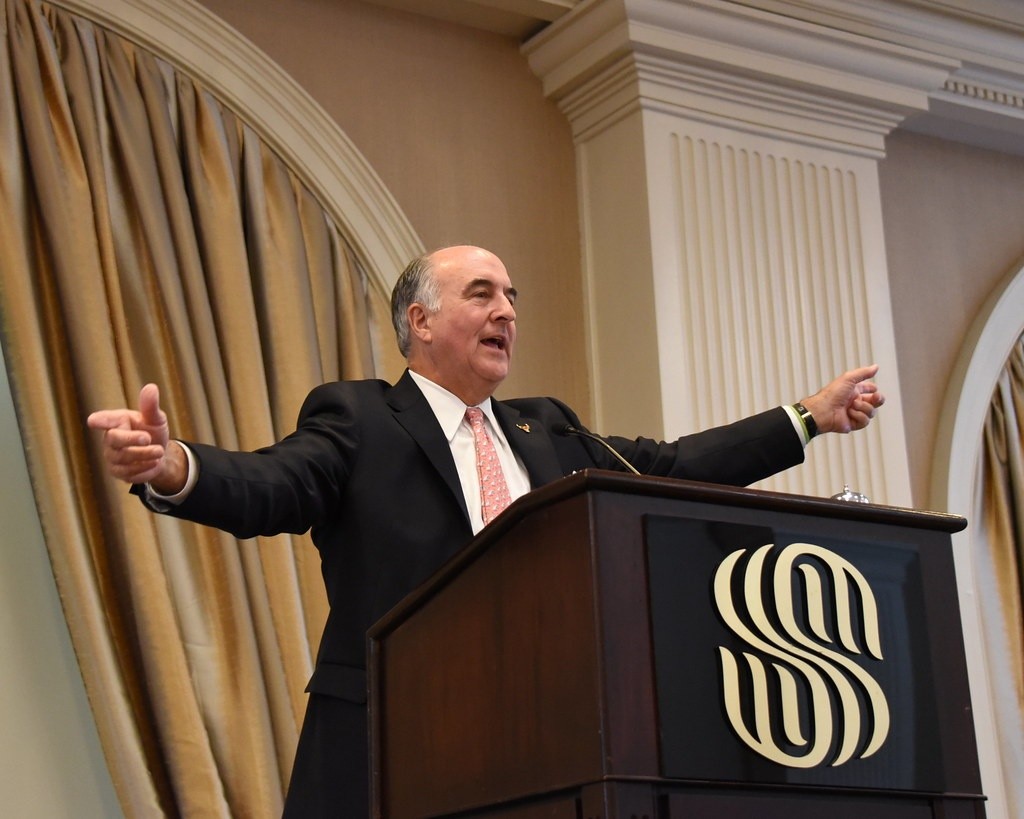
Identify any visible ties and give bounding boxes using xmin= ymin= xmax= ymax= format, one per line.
xmin=467 ymin=407 xmax=511 ymax=526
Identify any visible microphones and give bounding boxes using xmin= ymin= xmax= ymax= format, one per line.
xmin=549 ymin=421 xmax=643 ymax=482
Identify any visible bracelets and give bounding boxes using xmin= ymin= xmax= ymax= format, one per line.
xmin=792 ymin=403 xmax=820 ymax=439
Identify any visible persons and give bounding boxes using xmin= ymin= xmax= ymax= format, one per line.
xmin=78 ymin=244 xmax=891 ymax=817
